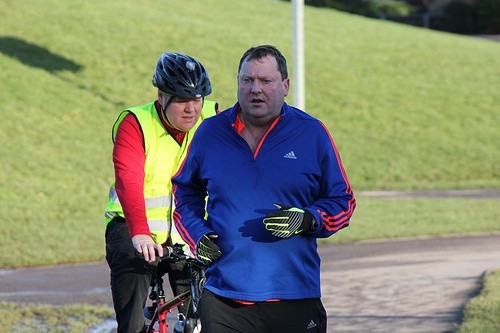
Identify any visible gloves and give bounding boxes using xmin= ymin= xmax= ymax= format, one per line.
xmin=197 ymin=234 xmax=220 ymax=265
xmin=263 ymin=203 xmax=312 ymax=237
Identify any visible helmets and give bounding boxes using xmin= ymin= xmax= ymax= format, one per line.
xmin=152 ymin=52 xmax=212 ymax=99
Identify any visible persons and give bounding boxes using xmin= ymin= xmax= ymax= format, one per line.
xmin=170 ymin=44 xmax=357 ymax=333
xmin=103 ymin=52 xmax=221 ymax=333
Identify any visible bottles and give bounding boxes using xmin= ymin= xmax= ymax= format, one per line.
xmin=173 ymin=313 xmax=186 ymax=333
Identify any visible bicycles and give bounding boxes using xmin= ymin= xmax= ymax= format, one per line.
xmin=133 ymin=245 xmax=218 ymax=332
